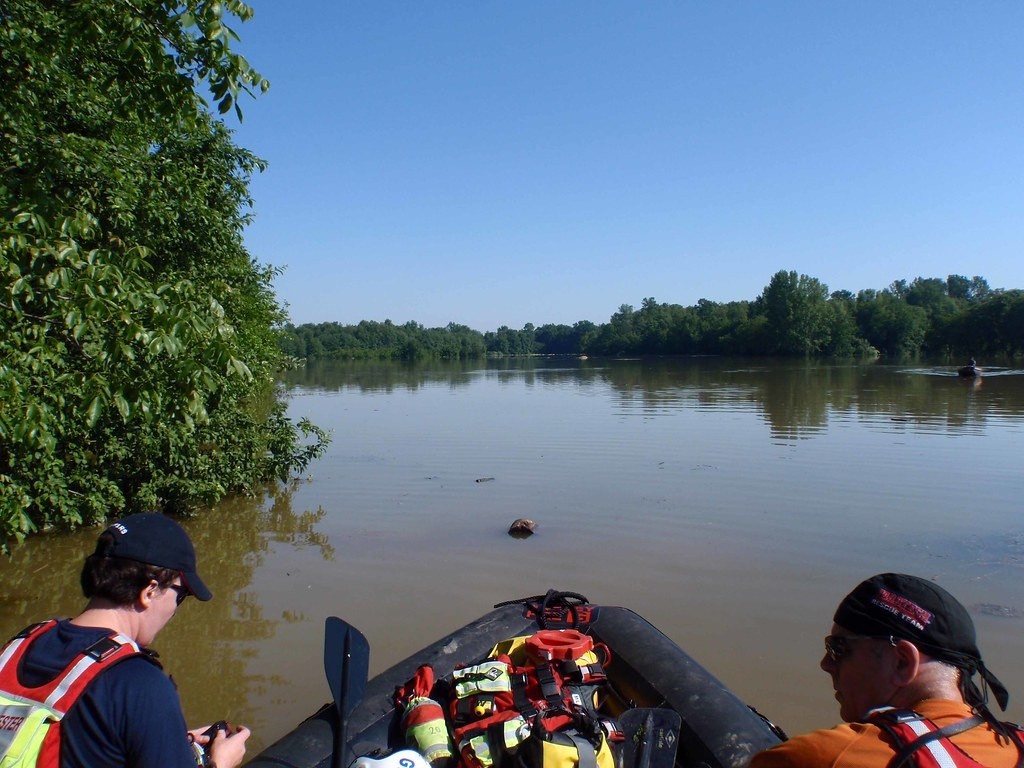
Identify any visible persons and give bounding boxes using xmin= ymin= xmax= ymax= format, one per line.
xmin=0 ymin=511 xmax=250 ymax=768
xmin=749 ymin=573 xmax=1024 ymax=768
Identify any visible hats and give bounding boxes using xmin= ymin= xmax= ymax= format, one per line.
xmin=96 ymin=511 xmax=213 ymax=601
xmin=833 ymin=573 xmax=1009 ymax=713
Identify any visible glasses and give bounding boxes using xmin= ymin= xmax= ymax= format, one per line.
xmin=163 ymin=583 xmax=189 ymax=607
xmin=824 ymin=635 xmax=901 ymax=665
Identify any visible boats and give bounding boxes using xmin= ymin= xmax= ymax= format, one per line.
xmin=579 ymin=356 xmax=588 ymax=359
xmin=957 ymin=367 xmax=976 ymax=377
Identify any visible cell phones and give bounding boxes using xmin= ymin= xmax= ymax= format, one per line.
xmin=198 ymin=719 xmax=232 ymax=751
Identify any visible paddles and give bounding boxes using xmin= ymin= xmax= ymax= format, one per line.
xmin=614 ymin=706 xmax=684 ymax=768
xmin=320 ymin=615 xmax=371 ymax=768
